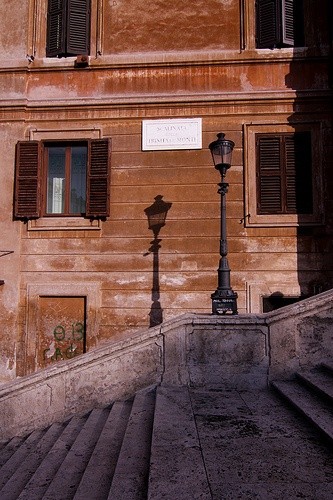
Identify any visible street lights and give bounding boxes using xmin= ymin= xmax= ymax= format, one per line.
xmin=208 ymin=133 xmax=238 ymax=315
